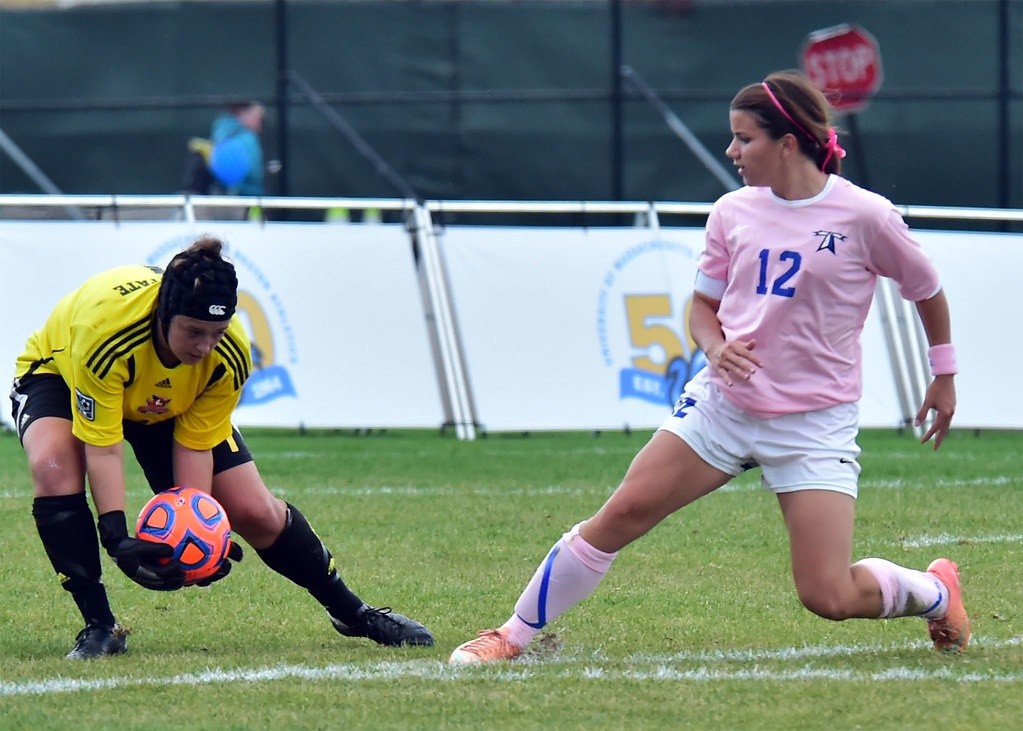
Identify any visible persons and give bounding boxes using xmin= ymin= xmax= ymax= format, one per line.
xmin=448 ymin=71 xmax=968 ymax=663
xmin=11 ymin=238 xmax=434 ymax=660
xmin=187 ymin=97 xmax=284 ymax=198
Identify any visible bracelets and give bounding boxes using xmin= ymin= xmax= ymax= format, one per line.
xmin=928 ymin=343 xmax=958 ymax=374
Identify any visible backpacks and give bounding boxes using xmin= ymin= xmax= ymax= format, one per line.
xmin=183 ymin=128 xmax=243 ymax=194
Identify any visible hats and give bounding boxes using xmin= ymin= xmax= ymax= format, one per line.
xmin=158 ymin=252 xmax=238 ymax=350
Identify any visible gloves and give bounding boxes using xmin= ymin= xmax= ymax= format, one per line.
xmin=98 ymin=510 xmax=187 ymax=591
xmin=184 ymin=541 xmax=243 ymax=587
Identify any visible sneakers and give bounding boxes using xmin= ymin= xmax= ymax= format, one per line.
xmin=325 ymin=602 xmax=437 ymax=647
xmin=450 ymin=629 xmax=522 ymax=667
xmin=926 ymin=557 xmax=970 ymax=654
xmin=64 ymin=622 xmax=128 ymax=659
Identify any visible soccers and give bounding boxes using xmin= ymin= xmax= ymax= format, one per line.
xmin=136 ymin=486 xmax=231 ymax=584
